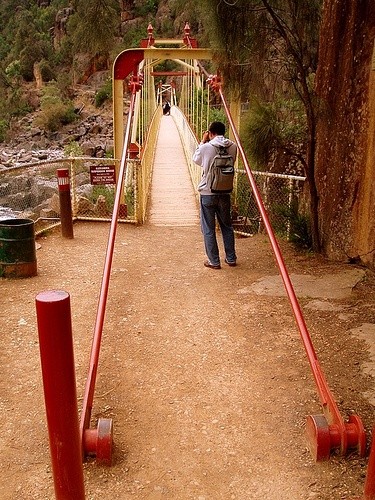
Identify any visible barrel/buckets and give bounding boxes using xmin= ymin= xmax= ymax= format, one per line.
xmin=0 ymin=218 xmax=37 ymax=279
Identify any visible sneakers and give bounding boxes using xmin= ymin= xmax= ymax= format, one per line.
xmin=225 ymin=258 xmax=236 ymax=266
xmin=204 ymin=259 xmax=221 ymax=269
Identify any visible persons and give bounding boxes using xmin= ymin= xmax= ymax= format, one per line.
xmin=192 ymin=121 xmax=237 ymax=270
xmin=162 ymin=102 xmax=171 ymax=115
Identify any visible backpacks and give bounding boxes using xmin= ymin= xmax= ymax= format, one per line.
xmin=210 ymin=146 xmax=234 ymax=193
